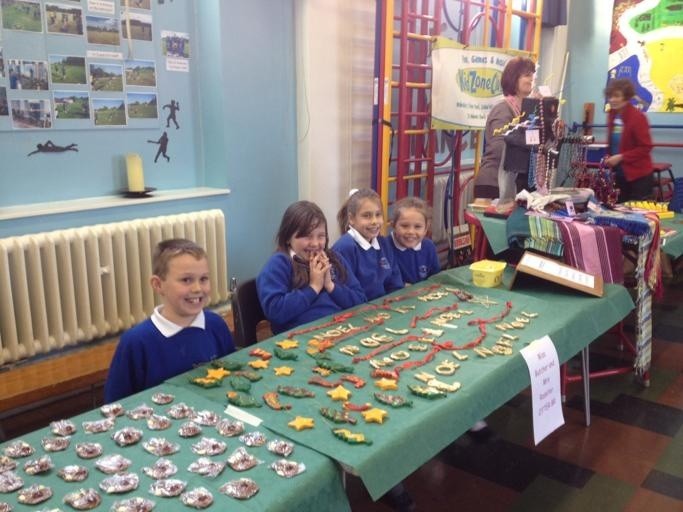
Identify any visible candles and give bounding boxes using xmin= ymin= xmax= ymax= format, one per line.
xmin=126 ymin=153 xmax=145 ymax=192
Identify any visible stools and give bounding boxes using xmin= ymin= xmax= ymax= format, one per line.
xmin=570 ymin=158 xmax=675 ymax=203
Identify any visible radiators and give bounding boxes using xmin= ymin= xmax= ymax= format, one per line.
xmin=0 ymin=209 xmax=238 ymax=366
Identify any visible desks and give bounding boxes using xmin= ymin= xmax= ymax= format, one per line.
xmin=163 ymin=258 xmax=636 ymax=505
xmin=463 ymin=207 xmax=664 ymax=405
xmin=0 ymin=384 xmax=355 ymax=512
xmin=660 ymin=213 xmax=683 ymax=262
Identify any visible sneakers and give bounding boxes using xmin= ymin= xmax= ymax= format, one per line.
xmin=387 ymin=483 xmax=415 ymax=512
xmin=468 ymin=421 xmax=495 ymax=443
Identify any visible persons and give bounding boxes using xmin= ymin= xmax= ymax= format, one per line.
xmin=96 ymin=238 xmax=238 ymax=408
xmin=254 ymin=199 xmax=365 ymax=337
xmin=597 ymin=78 xmax=657 ymax=204
xmin=325 ymin=186 xmax=403 ymax=300
xmin=378 ymin=195 xmax=441 ymax=286
xmin=0 ymin=1 xmax=187 ymax=131
xmin=472 ymin=56 xmax=536 ymax=201
